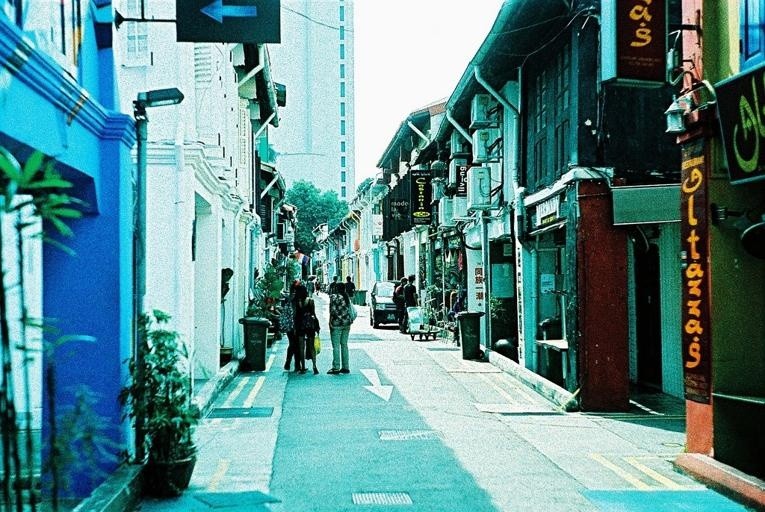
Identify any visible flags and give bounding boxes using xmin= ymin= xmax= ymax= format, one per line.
xmin=294 ymin=250 xmax=310 ymax=264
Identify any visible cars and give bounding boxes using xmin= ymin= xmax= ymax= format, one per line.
xmin=367 ymin=280 xmax=403 ymax=329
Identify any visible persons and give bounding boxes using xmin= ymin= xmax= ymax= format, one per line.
xmin=221 ymin=267 xmax=233 ymax=349
xmin=263 ymin=274 xmax=356 ymax=374
xmin=393 ymin=275 xmax=420 ymax=334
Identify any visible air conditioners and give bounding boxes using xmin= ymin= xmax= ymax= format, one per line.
xmin=438 ymin=93 xmax=492 ymax=228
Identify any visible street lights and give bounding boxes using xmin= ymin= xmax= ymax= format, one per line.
xmin=132 ymin=84 xmax=187 ymax=379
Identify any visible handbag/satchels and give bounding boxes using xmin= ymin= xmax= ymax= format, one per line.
xmin=303 ymin=312 xmax=314 ymax=330
xmin=348 ymin=302 xmax=358 ymax=320
xmin=306 ymin=336 xmax=320 ymax=359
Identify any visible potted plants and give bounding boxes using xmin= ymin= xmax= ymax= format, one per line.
xmin=244 ymin=261 xmax=284 ymax=349
xmin=115 ymin=310 xmax=207 ymax=499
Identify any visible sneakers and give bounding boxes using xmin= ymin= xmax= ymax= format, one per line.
xmin=284 ymin=360 xmax=319 ymax=373
xmin=327 ymin=368 xmax=350 ymax=374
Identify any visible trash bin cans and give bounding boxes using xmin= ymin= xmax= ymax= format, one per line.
xmin=239 ymin=317 xmax=272 ymax=372
xmin=455 ymin=310 xmax=485 ymax=360
xmin=351 ymin=289 xmax=367 ymax=306
